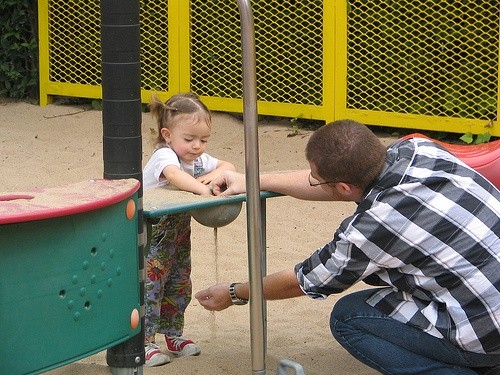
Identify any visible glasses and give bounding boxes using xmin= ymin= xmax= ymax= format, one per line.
xmin=308 ymin=172 xmax=352 ymax=187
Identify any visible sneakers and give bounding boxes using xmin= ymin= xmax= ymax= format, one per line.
xmin=165 ymin=334 xmax=201 ymax=356
xmin=144 ymin=343 xmax=170 ymax=366
